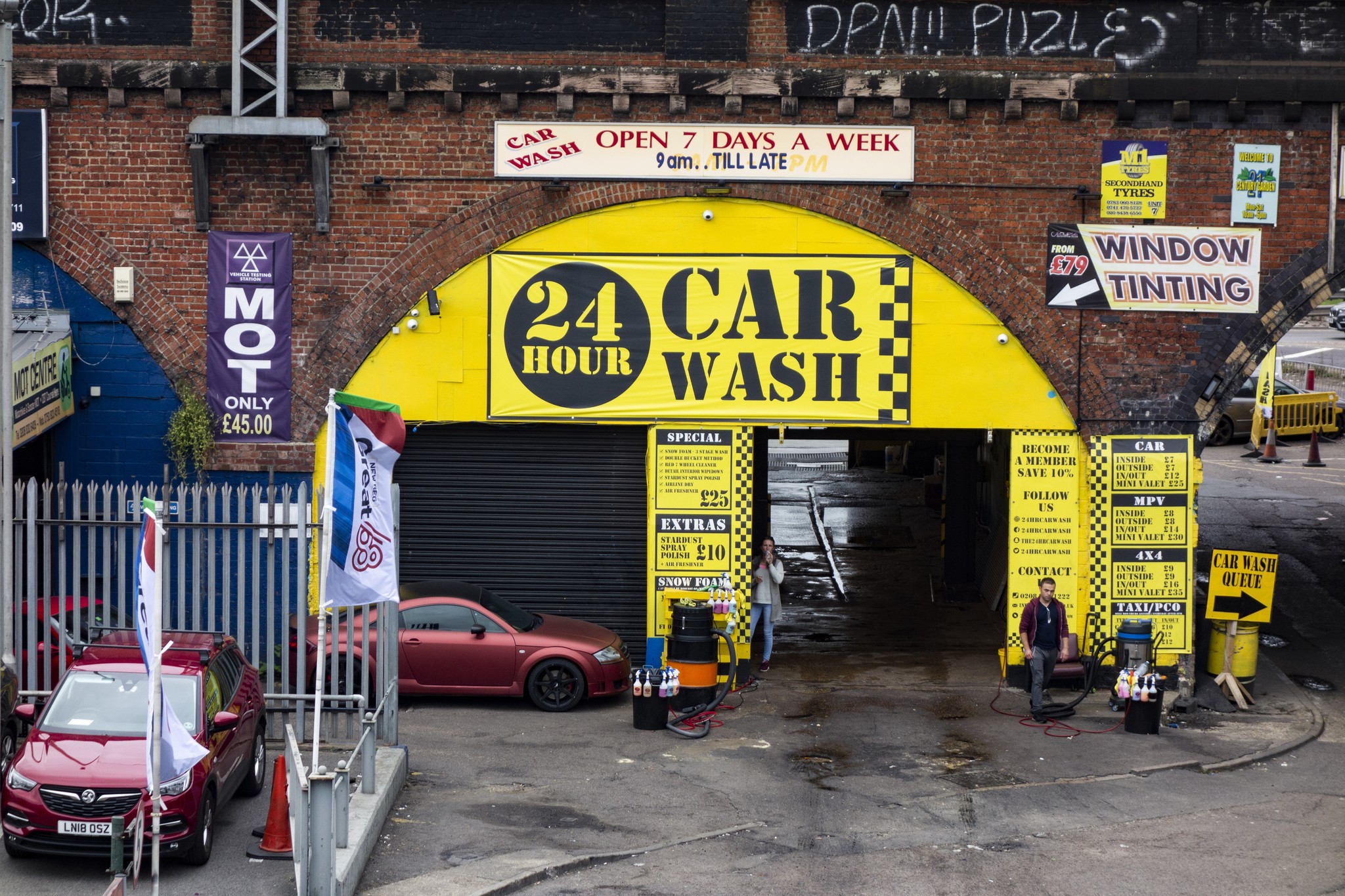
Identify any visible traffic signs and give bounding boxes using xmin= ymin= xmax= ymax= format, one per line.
xmin=1204 ymin=549 xmax=1280 ymax=623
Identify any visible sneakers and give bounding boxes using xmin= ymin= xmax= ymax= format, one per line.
xmin=1032 ymin=713 xmax=1047 ymax=723
xmin=760 ymin=661 xmax=770 ymax=672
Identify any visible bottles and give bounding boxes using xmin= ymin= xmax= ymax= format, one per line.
xmin=765 ymin=550 xmax=771 ymax=561
xmin=1134 ymin=661 xmax=1149 ymax=677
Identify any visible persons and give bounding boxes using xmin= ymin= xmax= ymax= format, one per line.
xmin=1019 ymin=577 xmax=1069 ymax=723
xmin=750 ymin=536 xmax=784 ymax=671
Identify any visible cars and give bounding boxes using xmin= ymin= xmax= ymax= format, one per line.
xmin=1204 ymin=369 xmax=1345 ymax=447
xmin=285 ymin=576 xmax=634 ymax=713
xmin=10 ymin=596 xmax=136 ymax=697
xmin=1327 ymin=300 xmax=1345 ymax=333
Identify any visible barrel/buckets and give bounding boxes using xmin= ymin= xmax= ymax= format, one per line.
xmin=1207 ymin=619 xmax=1260 ymax=702
xmin=998 ymin=648 xmax=1006 ymax=677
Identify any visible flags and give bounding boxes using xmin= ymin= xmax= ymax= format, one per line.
xmin=324 ymin=390 xmax=407 ymax=609
xmin=133 ymin=498 xmax=211 ymax=794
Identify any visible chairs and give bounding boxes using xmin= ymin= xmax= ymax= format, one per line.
xmin=1023 ymin=632 xmax=1087 ymax=692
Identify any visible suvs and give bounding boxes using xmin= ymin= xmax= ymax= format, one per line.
xmin=0 ymin=622 xmax=266 ymax=869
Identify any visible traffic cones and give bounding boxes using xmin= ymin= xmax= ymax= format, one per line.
xmin=1302 ymin=425 xmax=1327 ymax=467
xmin=245 ymin=752 xmax=295 ymax=860
xmin=1259 ymin=419 xmax=1286 ymax=462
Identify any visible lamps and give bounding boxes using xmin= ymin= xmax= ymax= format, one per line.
xmin=1072 ymin=186 xmax=1103 ymax=200
xmin=541 ymin=177 xmax=570 ymax=191
xmin=704 ymin=180 xmax=732 ymax=194
xmin=1199 ymin=373 xmax=1224 ymax=403
xmin=881 ymin=182 xmax=910 ymax=197
xmin=362 ymin=176 xmax=391 ymax=191
